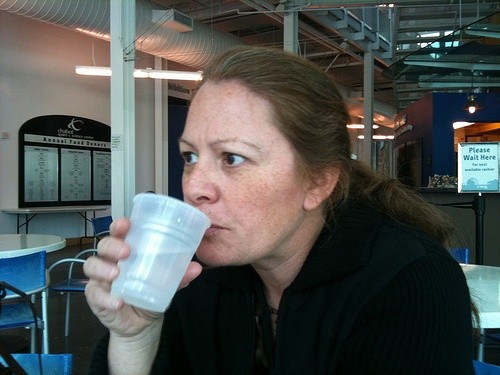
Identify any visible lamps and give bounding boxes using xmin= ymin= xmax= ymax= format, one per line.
xmin=464 ymin=70 xmax=482 ymax=113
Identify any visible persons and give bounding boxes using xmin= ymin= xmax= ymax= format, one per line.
xmin=83 ymin=46 xmax=476 ymax=375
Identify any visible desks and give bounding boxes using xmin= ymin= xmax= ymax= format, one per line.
xmin=2 ymin=208 xmax=107 ymax=244
xmin=460 ymin=260 xmax=500 ymax=362
xmin=0 ymin=231 xmax=67 ymax=354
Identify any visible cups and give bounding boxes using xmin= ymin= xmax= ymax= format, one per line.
xmin=109 ymin=193 xmax=212 ymax=314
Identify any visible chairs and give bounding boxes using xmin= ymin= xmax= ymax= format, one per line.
xmin=0 ymin=250 xmax=50 ymax=354
xmin=48 ymin=249 xmax=100 ymax=336
xmin=91 ymin=216 xmax=113 ymax=258
